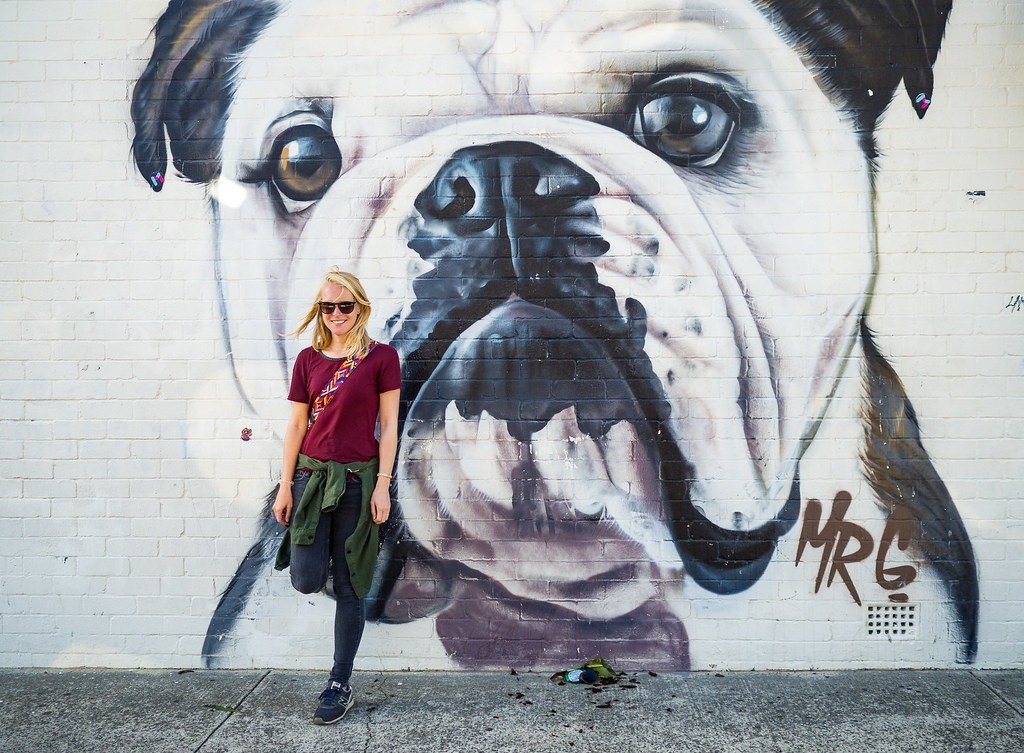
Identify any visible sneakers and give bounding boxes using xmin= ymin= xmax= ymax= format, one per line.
xmin=311 ymin=680 xmax=356 ymax=726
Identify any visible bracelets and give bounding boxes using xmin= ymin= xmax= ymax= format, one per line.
xmin=278 ymin=480 xmax=294 ymax=486
xmin=377 ymin=473 xmax=392 ymax=479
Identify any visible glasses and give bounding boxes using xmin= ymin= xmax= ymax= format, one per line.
xmin=321 ymin=301 xmax=357 ymax=315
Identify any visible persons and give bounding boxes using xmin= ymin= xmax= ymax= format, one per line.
xmin=273 ymin=264 xmax=401 ymax=726
